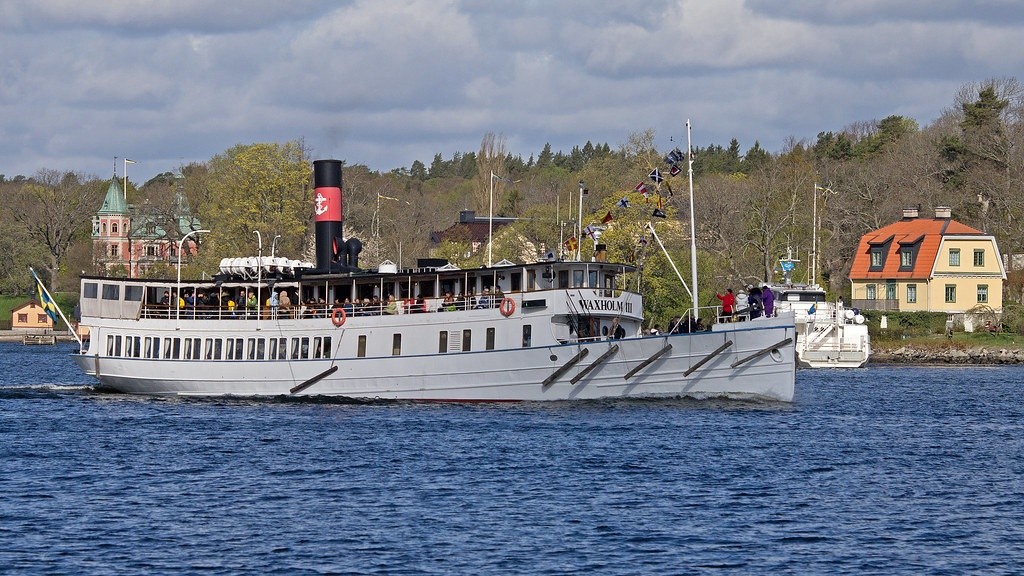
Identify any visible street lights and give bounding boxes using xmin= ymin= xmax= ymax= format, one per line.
xmin=253 ymin=230 xmax=261 ymax=330
xmin=272 ymin=235 xmax=281 ymax=257
xmin=176 ymin=229 xmax=210 ymax=329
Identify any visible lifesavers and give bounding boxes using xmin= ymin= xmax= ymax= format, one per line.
xmin=500 ymin=298 xmax=515 ymax=316
xmin=332 ymin=308 xmax=346 ymax=326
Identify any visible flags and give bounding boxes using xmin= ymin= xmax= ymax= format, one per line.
xmin=34 ymin=276 xmax=59 ymax=326
xmin=544 ymin=149 xmax=684 ymax=257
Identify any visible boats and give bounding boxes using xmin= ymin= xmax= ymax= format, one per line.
xmin=732 ymin=183 xmax=871 ymax=368
xmin=68 ymin=119 xmax=798 ymax=405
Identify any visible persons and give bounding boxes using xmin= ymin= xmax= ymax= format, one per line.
xmin=566 ymin=321 xmax=578 ymax=344
xmin=160 ymin=289 xmax=426 ymax=319
xmin=608 ymin=318 xmax=622 ymax=339
xmin=945 ymin=325 xmax=953 ymax=343
xmin=442 ymin=285 xmax=505 ymax=310
xmin=641 ymin=285 xmax=775 ymax=336
xmin=985 ymin=321 xmax=1002 ymax=333
